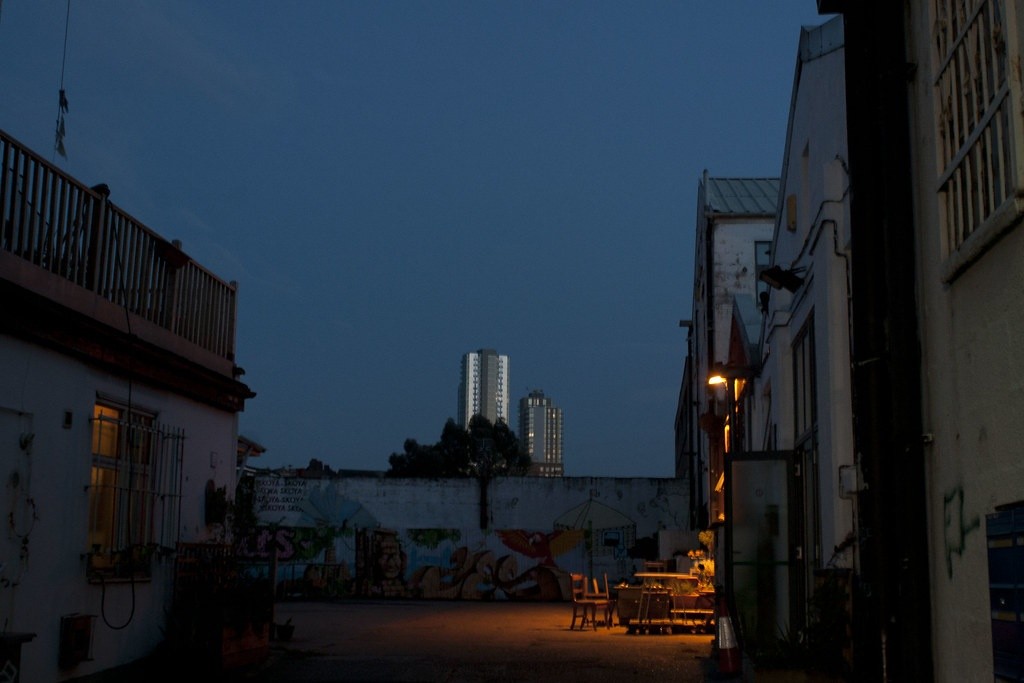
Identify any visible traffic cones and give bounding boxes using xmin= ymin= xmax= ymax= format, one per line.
xmin=714 ymin=586 xmax=745 ymax=681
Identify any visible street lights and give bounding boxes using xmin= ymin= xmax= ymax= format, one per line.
xmin=707 ymin=360 xmax=738 ymax=452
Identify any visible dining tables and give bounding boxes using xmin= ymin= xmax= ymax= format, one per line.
xmin=633 ymin=572 xmax=694 ymax=625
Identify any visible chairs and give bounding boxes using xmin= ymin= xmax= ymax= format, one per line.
xmin=570 ymin=571 xmax=619 ymax=632
